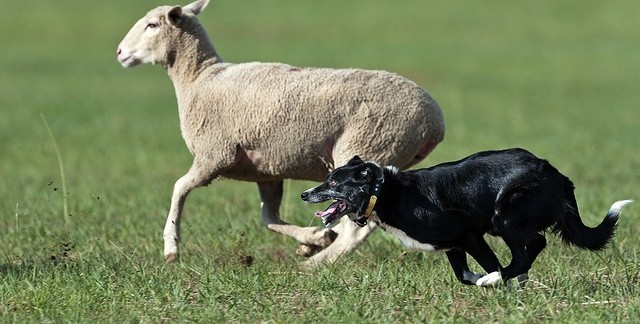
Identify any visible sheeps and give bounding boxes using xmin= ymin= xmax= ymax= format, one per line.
xmin=116 ymin=0 xmax=446 ymax=271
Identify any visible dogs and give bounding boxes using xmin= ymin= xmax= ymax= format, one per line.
xmin=300 ymin=146 xmax=635 ymax=288
